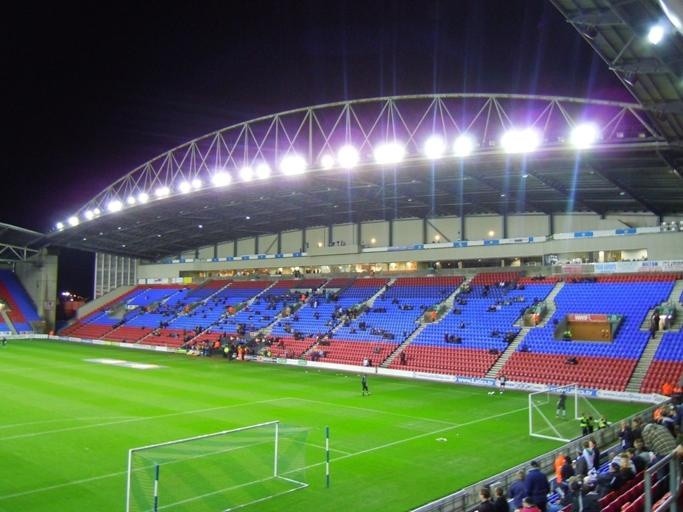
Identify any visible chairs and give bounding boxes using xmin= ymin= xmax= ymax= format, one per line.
xmin=557 ymin=460 xmax=682 ymax=511
xmin=0 ymin=269 xmax=683 ymax=404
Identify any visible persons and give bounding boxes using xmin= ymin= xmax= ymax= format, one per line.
xmin=361 ymin=373 xmax=371 ymax=397
xmin=555 ymin=390 xmax=566 ymax=416
xmin=498 ymin=372 xmax=506 ymax=392
xmin=138 ymin=264 xmax=660 ymax=366
xmin=472 ymin=396 xmax=683 ymax=512
